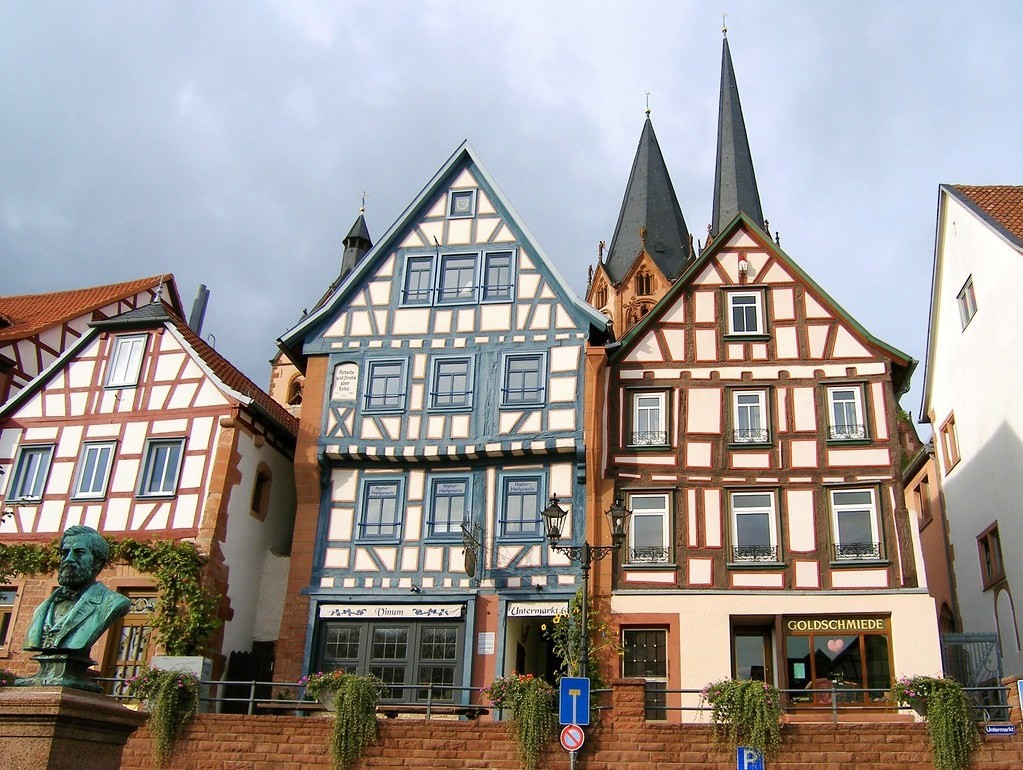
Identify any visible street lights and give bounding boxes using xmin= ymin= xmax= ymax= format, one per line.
xmin=539 ymin=492 xmax=633 ymax=770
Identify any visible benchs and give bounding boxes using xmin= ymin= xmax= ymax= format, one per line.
xmin=257 ymin=703 xmax=490 ymax=722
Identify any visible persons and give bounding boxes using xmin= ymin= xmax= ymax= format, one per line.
xmin=23 ymin=525 xmax=131 ymax=649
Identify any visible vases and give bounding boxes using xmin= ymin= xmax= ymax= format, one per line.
xmin=317 ymin=689 xmax=338 ymax=711
xmin=907 ymin=702 xmax=928 ymax=717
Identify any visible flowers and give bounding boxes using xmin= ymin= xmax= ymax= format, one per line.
xmin=292 ymin=667 xmax=390 ymax=770
xmin=885 ymin=672 xmax=984 ymax=770
xmin=694 ymin=674 xmax=789 ymax=767
xmin=475 ymin=670 xmax=559 ymax=770
xmin=124 ymin=664 xmax=208 ymax=770
